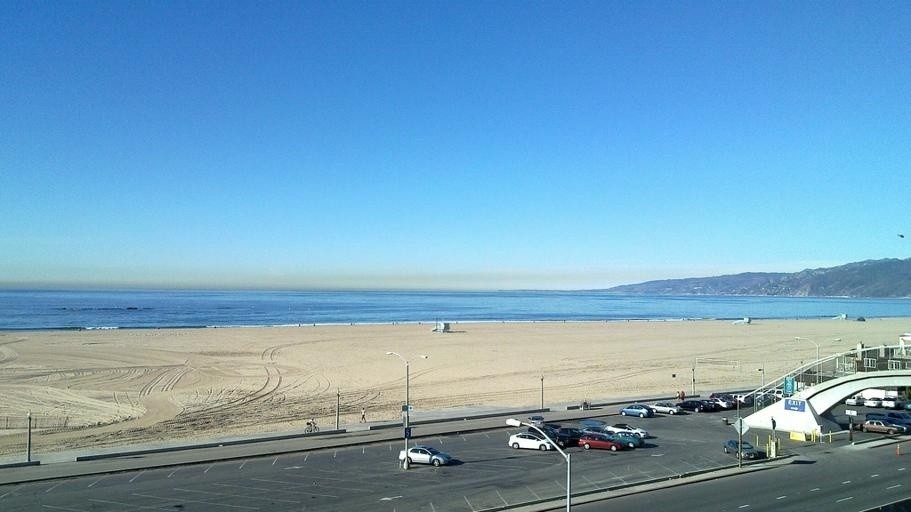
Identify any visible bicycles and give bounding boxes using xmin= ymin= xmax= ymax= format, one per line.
xmin=304 ymin=424 xmax=319 ymax=434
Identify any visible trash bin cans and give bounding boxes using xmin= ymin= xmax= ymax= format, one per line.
xmin=723 ymin=417 xmax=728 ymax=425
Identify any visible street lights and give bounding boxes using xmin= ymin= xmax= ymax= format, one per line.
xmin=506 ymin=419 xmax=571 ymax=512
xmin=386 ymin=352 xmax=428 ymax=469
xmin=795 ymin=337 xmax=841 ymax=383
xmin=336 ymin=390 xmax=340 ymax=429
xmin=540 ymin=374 xmax=544 ymax=408
xmin=27 ymin=413 xmax=31 ymax=460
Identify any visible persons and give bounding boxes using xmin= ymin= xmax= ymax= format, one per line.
xmin=309 ymin=417 xmax=317 ymax=431
xmin=676 ymin=390 xmax=685 ymax=403
xmin=359 ymin=407 xmax=366 ymax=423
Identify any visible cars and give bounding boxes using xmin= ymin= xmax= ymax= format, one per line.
xmin=399 ymin=446 xmax=452 ymax=466
xmin=724 ymin=440 xmax=758 ymax=460
xmin=863 ymin=413 xmax=911 ymax=434
xmin=619 ymin=389 xmax=783 ymax=418
xmin=508 ymin=424 xmax=649 ymax=451
xmin=846 ymin=395 xmax=911 ymax=410
xmin=528 ymin=416 xmax=544 ymax=424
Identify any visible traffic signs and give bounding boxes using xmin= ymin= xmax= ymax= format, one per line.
xmin=785 ymin=399 xmax=805 ymax=412
xmin=405 ymin=428 xmax=411 ymax=438
